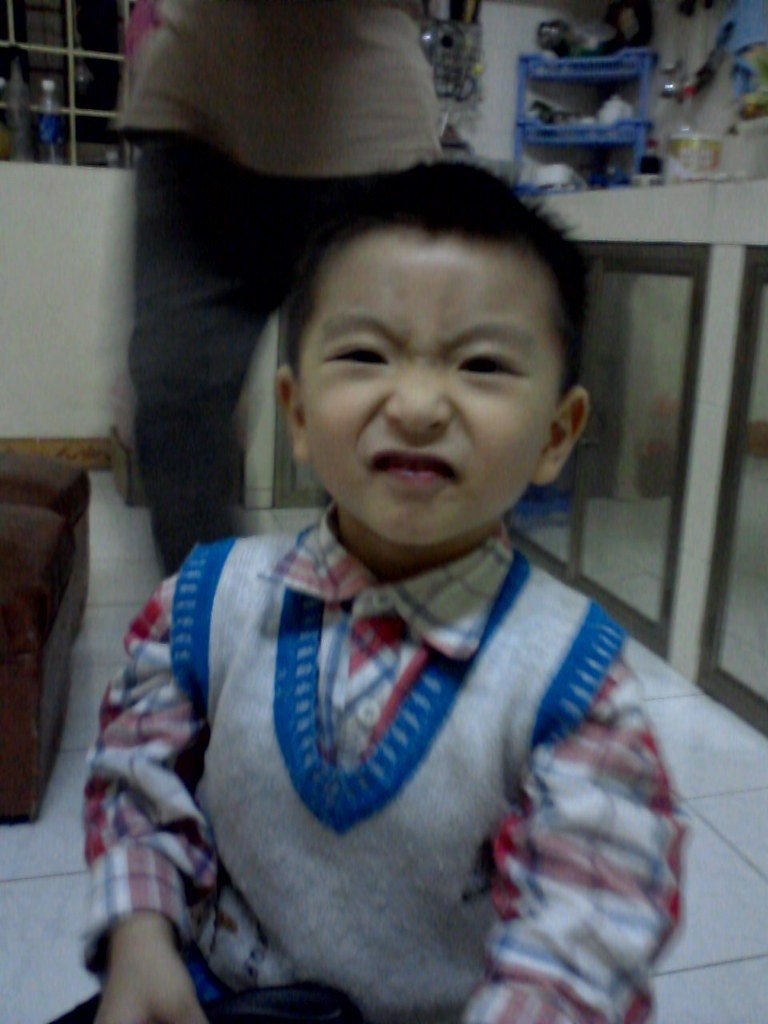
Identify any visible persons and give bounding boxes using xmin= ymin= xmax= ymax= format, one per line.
xmin=42 ymin=157 xmax=691 ymax=1024
xmin=113 ymin=0 xmax=450 ymax=579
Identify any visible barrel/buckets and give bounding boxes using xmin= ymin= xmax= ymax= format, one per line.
xmin=665 ymin=134 xmax=723 ymax=186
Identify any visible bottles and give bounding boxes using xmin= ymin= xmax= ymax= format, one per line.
xmin=7 ymin=40 xmax=33 ymax=163
xmin=34 ymin=76 xmax=65 ymax=164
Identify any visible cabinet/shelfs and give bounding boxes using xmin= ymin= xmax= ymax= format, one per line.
xmin=511 ymin=45 xmax=654 ymax=195
